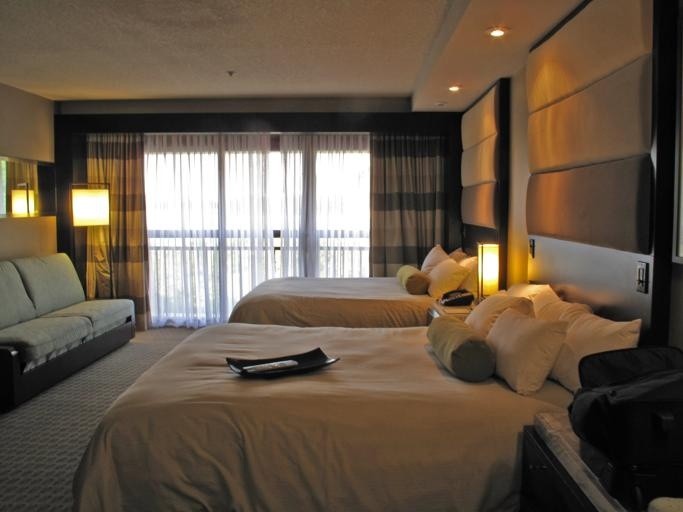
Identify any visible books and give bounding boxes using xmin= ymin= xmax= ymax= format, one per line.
xmin=443 ymin=308 xmax=470 ymax=314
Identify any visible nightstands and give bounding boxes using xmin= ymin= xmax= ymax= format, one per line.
xmin=522 ymin=424 xmax=652 ymax=512
xmin=428 ymin=301 xmax=472 ymax=324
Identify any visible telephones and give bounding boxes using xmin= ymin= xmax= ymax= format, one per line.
xmin=441 ymin=289 xmax=474 ymax=306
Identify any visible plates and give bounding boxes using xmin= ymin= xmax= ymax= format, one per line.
xmin=221 ymin=345 xmax=336 ymax=380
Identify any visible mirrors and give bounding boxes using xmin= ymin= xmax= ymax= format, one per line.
xmin=1 ymin=154 xmax=67 ymax=254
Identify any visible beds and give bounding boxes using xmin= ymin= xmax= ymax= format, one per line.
xmin=72 ymin=324 xmax=682 ymax=512
xmin=229 ymin=277 xmax=508 ymax=325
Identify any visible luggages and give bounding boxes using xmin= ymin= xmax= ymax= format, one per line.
xmin=568 ymin=344 xmax=683 ymax=511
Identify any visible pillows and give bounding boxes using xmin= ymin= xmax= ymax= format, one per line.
xmin=398 ymin=244 xmax=478 ymax=295
xmin=466 ymin=290 xmax=532 ymax=339
xmin=486 ymin=308 xmax=568 ymax=395
xmin=550 ymin=303 xmax=643 ymax=394
xmin=427 ymin=315 xmax=495 ymax=383
xmin=508 ymin=283 xmax=552 ymax=304
xmin=534 ymin=287 xmax=591 ymax=322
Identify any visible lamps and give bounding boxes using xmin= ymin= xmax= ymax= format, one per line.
xmin=476 ymin=240 xmax=500 ymax=305
xmin=69 ymin=181 xmax=114 ymax=299
xmin=8 ymin=180 xmax=38 ymax=219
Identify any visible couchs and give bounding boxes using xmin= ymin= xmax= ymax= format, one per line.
xmin=0 ymin=252 xmax=136 ymax=409
